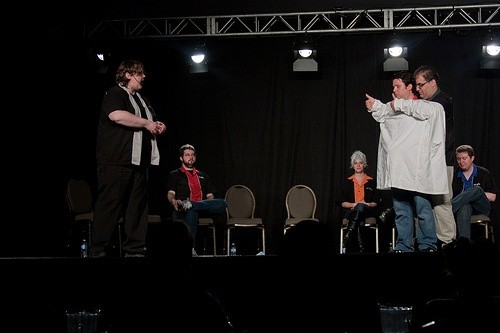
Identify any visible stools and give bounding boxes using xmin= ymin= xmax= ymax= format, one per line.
xmin=470 ymin=214 xmax=495 ymax=243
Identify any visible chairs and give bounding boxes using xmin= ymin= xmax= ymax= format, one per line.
xmin=340 ymin=218 xmax=379 ymax=254
xmin=65 ymin=177 xmax=216 ymax=255
xmin=224 ymin=185 xmax=266 ymax=256
xmin=283 ymin=184 xmax=319 ymax=236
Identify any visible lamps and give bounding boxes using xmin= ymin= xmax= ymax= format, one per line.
xmin=96 ymin=48 xmax=112 ymax=64
xmin=480 ymin=26 xmax=500 ymax=69
xmin=293 ymin=32 xmax=319 ymax=72
xmin=383 ymin=29 xmax=409 ymax=72
xmin=189 ymin=37 xmax=208 ymax=73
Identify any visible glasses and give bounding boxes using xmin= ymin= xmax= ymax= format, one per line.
xmin=415 ymin=78 xmax=434 ymax=88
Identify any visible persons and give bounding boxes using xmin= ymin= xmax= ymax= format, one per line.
xmin=340 ymin=151 xmax=379 ymax=254
xmin=90 ymin=58 xmax=167 ymax=258
xmin=451 ymin=145 xmax=496 ymax=243
xmin=365 ymin=67 xmax=455 ymax=254
xmin=167 ymin=144 xmax=227 ymax=256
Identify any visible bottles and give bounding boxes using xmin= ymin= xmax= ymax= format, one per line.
xmin=80 ymin=239 xmax=88 ymax=258
xmin=230 ymin=243 xmax=237 ymax=256
xmin=387 ymin=242 xmax=395 ymax=252
xmin=342 ymin=243 xmax=346 ymax=254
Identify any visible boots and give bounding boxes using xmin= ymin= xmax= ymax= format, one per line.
xmin=355 ymin=226 xmax=365 ymax=254
xmin=342 ymin=209 xmax=363 ymax=243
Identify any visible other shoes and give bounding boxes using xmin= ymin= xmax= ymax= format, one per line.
xmin=392 ymin=248 xmax=402 ymax=253
xmin=421 ymin=248 xmax=436 ymax=253
xmin=192 ymin=248 xmax=197 ymax=255
xmin=182 ymin=200 xmax=192 ymax=212
xmin=89 ymin=250 xmax=105 ymax=258
xmin=123 ymin=251 xmax=146 ymax=257
